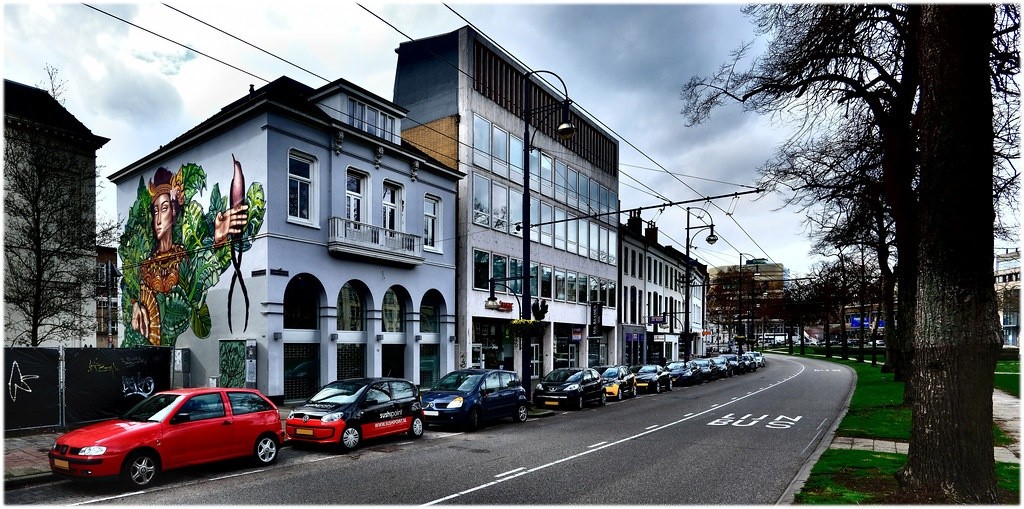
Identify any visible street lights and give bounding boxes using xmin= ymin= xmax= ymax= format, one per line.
xmin=522 ymin=69 xmax=575 ymax=411
xmin=684 ymin=208 xmax=719 ymax=363
xmin=739 ymin=253 xmax=768 ymax=355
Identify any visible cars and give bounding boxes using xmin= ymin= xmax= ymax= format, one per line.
xmin=754 ymin=330 xmax=884 ymax=347
xmin=628 ymin=364 xmax=672 ymax=394
xmin=285 ymin=376 xmax=424 ymax=452
xmin=48 ymin=387 xmax=286 ymax=491
xmin=422 ymin=369 xmax=528 ymax=432
xmin=664 ymin=362 xmax=703 ymax=387
xmin=688 ymin=346 xmax=765 ymax=382
xmin=592 ymin=365 xmax=637 ymax=402
xmin=533 ymin=367 xmax=606 ymax=411
